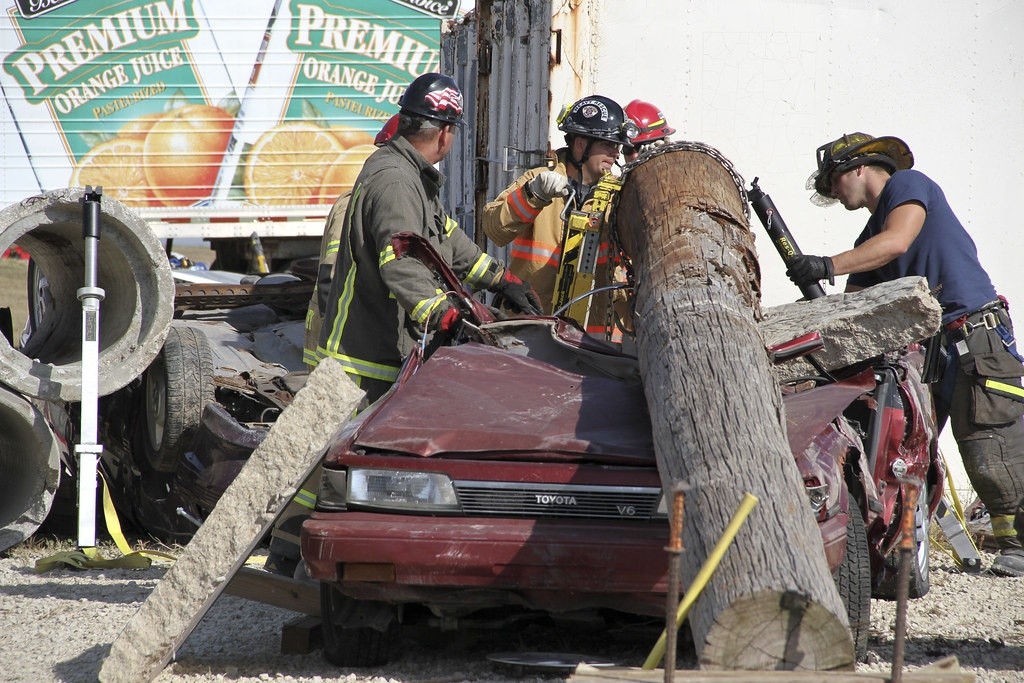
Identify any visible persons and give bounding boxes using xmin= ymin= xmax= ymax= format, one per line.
xmin=611 ymin=100 xmax=684 ymax=337
xmin=264 ymin=113 xmax=471 ymax=585
xmin=478 ymin=94 xmax=644 ymax=361
xmin=782 ymin=129 xmax=1024 ymax=575
xmin=314 ymin=69 xmax=546 ymax=443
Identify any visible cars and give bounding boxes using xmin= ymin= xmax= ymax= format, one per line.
xmin=26 ymin=245 xmax=363 ymax=521
xmin=288 ymin=228 xmax=943 ymax=665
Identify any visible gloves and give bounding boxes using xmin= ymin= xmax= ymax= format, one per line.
xmin=437 ymin=308 xmax=475 ymax=343
xmin=487 ymin=267 xmax=544 ymax=316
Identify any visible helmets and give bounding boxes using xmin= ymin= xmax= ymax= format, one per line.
xmin=557 ymin=96 xmax=636 ymax=148
xmin=375 ymin=112 xmax=398 ymax=147
xmin=814 ymin=132 xmax=915 ymax=199
xmin=622 ymin=99 xmax=675 ymax=143
xmin=396 ymin=73 xmax=468 ymax=126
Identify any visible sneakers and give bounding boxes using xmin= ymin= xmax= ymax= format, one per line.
xmin=263 ymin=551 xmax=295 ymax=578
xmin=991 ymin=554 xmax=1024 ymax=576
